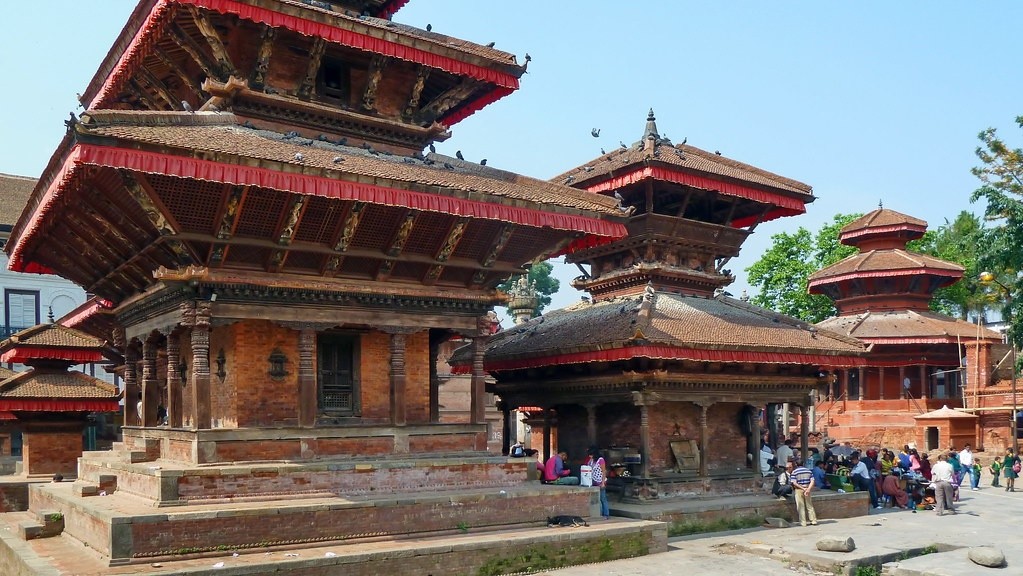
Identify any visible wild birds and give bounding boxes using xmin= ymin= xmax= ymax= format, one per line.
xmin=512 ymin=313 xmax=544 ymax=337
xmin=404 ymin=142 xmax=487 ymax=171
xmin=244 ymin=118 xmax=393 ymax=163
xmin=555 ymin=132 xmax=722 ymax=185
xmin=584 ymin=284 xmax=644 ymax=323
xmin=660 ymin=286 xmax=734 ymax=300
xmin=723 ymin=268 xmax=732 ymax=277
xmin=840 ymin=312 xmax=864 ymax=327
xmin=614 ymin=190 xmax=625 ymax=201
xmin=591 ymin=128 xmax=601 ymax=137
xmin=209 ymin=103 xmax=220 ymax=113
xmin=772 ymin=316 xmax=818 ymax=339
xmin=181 ymin=100 xmax=195 ymax=113
xmin=426 ymin=24 xmax=532 ymax=62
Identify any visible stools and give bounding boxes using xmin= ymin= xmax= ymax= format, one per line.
xmin=883 ymin=495 xmax=895 ymax=508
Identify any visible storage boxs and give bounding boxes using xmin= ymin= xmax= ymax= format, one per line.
xmin=825 ymin=474 xmax=853 ymax=491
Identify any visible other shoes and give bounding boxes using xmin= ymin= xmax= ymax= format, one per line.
xmin=810 ymin=520 xmax=818 ymax=524
xmin=876 ymin=506 xmax=882 ymax=509
xmin=780 ymin=496 xmax=786 ymax=500
xmin=786 ymin=494 xmax=792 ymax=496
xmin=948 ymin=510 xmax=955 ymax=515
xmin=991 ymin=483 xmax=995 ymax=486
xmin=935 ymin=512 xmax=943 ymax=516
xmin=796 ymin=523 xmax=806 ymax=526
xmin=995 ymin=484 xmax=999 ymax=487
xmin=973 ymin=487 xmax=978 ymax=490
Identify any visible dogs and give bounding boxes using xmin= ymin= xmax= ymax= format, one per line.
xmin=546 ymin=515 xmax=589 ymax=528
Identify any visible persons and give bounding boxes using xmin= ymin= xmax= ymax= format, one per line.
xmin=747 ymin=426 xmax=982 ymax=527
xmin=137 ymin=392 xmax=168 ymax=425
xmin=989 ymin=447 xmax=1021 ymax=492
xmin=904 ymin=374 xmax=911 ymax=398
xmin=522 ymin=446 xmax=609 ymax=520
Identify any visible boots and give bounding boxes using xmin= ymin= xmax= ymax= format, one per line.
xmin=1010 ymin=483 xmax=1014 ymax=491
xmin=1005 ymin=483 xmax=1009 ymax=491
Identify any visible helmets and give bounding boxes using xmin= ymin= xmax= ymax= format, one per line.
xmin=866 ymin=449 xmax=877 ymax=457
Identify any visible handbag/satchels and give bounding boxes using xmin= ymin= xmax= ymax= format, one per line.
xmin=1012 ymin=454 xmax=1021 ymax=473
